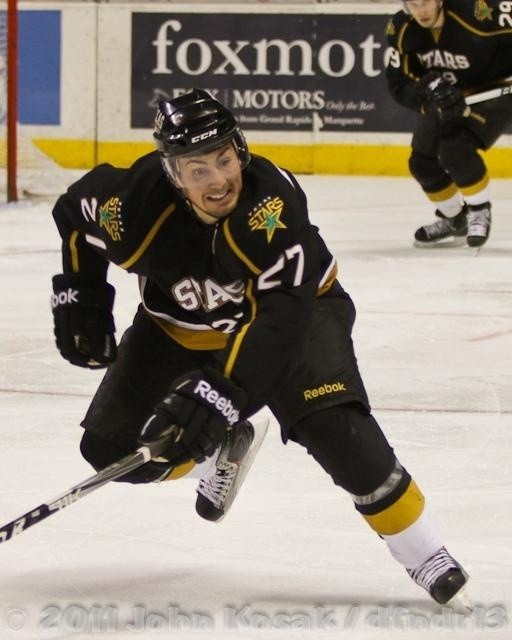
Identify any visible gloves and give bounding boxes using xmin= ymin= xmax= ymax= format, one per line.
xmin=48 ymin=273 xmax=118 ymax=370
xmin=134 ymin=363 xmax=250 ymax=470
xmin=415 ymin=69 xmax=468 ymax=131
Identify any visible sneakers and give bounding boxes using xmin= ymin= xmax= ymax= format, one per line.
xmin=413 ymin=200 xmax=468 ymax=242
xmin=196 ymin=420 xmax=256 ymax=522
xmin=405 ymin=543 xmax=465 ymax=604
xmin=467 ymin=201 xmax=493 ymax=248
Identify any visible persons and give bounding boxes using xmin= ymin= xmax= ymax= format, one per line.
xmin=50 ymin=89 xmax=472 ymax=606
xmin=383 ymin=0 xmax=511 ymax=246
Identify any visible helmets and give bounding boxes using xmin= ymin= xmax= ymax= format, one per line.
xmin=151 ymin=88 xmax=252 ymax=195
